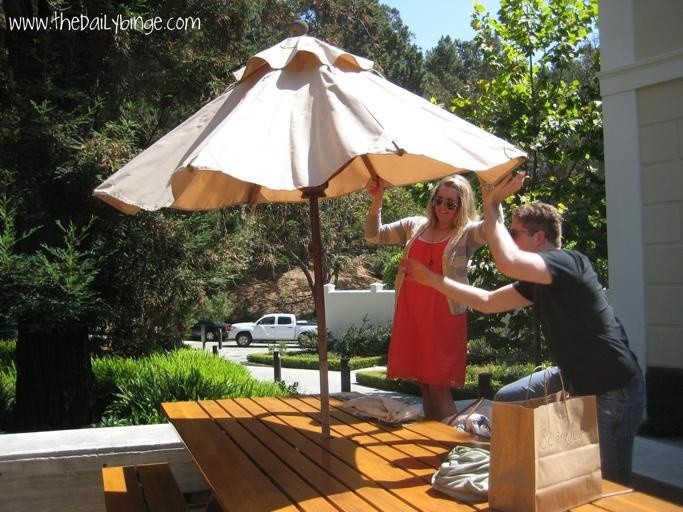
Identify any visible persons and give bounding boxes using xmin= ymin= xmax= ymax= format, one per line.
xmin=364 ymin=169 xmax=526 ymax=420
xmin=400 ymin=171 xmax=647 ymax=489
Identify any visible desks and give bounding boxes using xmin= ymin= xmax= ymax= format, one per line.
xmin=159 ymin=390 xmax=681 ymax=511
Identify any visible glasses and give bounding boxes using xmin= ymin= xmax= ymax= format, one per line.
xmin=510 ymin=229 xmax=537 ymax=239
xmin=432 ymin=197 xmax=458 ymax=211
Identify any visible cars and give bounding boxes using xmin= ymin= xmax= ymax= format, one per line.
xmin=185 ymin=319 xmax=230 ymax=341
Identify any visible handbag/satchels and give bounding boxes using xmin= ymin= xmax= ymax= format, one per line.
xmin=488 ymin=390 xmax=603 ymax=512
xmin=344 ymin=395 xmax=420 ymax=424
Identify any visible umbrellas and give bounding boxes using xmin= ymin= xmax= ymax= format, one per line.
xmin=92 ymin=21 xmax=531 ymax=442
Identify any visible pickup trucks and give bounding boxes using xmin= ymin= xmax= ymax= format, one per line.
xmin=226 ymin=312 xmax=321 ymax=348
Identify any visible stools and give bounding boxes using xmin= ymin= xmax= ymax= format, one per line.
xmin=98 ymin=460 xmax=188 ymax=511
